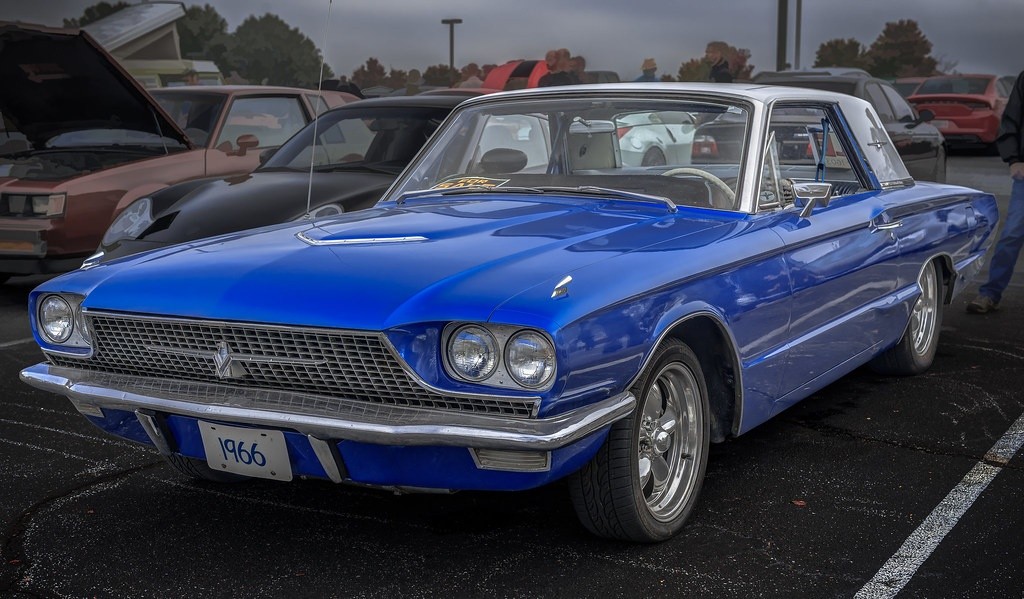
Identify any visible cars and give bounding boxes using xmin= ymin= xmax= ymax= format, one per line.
xmin=614 ymin=110 xmax=699 ymax=168
xmin=18 ymin=81 xmax=998 ymax=542
xmin=314 ymin=57 xmax=549 ymax=102
xmin=906 ymin=72 xmax=1019 ymax=155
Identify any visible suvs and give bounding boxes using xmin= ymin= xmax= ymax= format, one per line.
xmin=690 ymin=67 xmax=948 ymax=188
xmin=0 ymin=18 xmax=369 ymax=286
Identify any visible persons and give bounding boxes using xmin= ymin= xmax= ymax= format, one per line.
xmin=404 ymin=70 xmax=425 ymax=96
xmin=966 ymin=71 xmax=1024 ymax=314
xmin=634 ymin=58 xmax=667 ymax=82
xmin=538 ymin=49 xmax=585 ymax=87
xmin=705 ymin=42 xmax=733 ymax=83
xmin=451 ymin=62 xmax=497 ymax=88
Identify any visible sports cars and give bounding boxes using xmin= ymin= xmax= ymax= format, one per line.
xmin=80 ymin=94 xmax=480 ymax=269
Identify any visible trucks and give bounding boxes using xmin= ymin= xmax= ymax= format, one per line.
xmin=81 ymin=1 xmax=227 ymax=87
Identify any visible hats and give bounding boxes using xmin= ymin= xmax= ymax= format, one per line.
xmin=404 ymin=69 xmax=425 ymax=85
xmin=640 ymin=57 xmax=657 ymax=70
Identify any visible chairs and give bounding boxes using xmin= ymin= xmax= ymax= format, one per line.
xmin=716 ymin=175 xmax=795 ymax=209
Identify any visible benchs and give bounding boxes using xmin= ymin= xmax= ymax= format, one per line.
xmin=789 ymin=177 xmax=859 ymax=196
